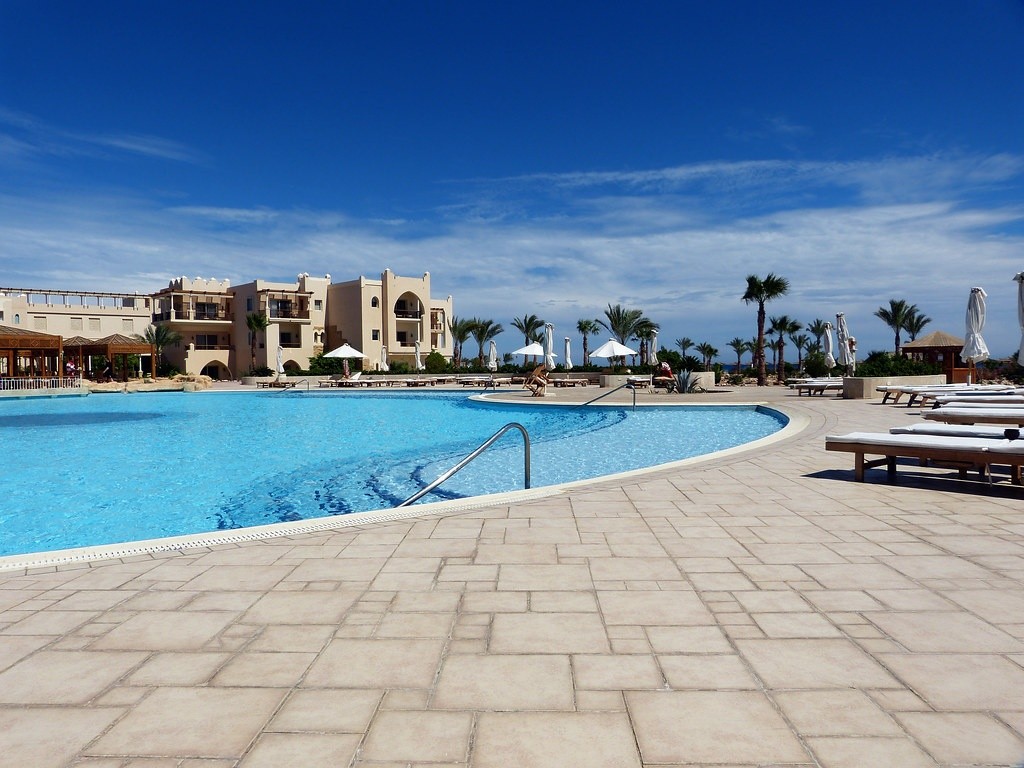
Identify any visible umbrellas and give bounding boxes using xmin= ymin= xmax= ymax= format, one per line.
xmin=276 ymin=345 xmax=285 ymax=380
xmin=542 ymin=323 xmax=557 ymax=380
xmin=511 ymin=342 xmax=558 ymax=373
xmin=822 ymin=322 xmax=837 ymax=379
xmin=564 ymin=337 xmax=573 ymax=380
xmin=1013 ymin=270 xmax=1024 ymax=370
xmin=487 ymin=340 xmax=497 ymax=380
xmin=960 ymin=286 xmax=990 ymax=364
xmin=835 ymin=312 xmax=854 ymax=377
xmin=380 ymin=344 xmax=389 ymax=372
xmin=648 ymin=329 xmax=659 ymax=376
xmin=414 ymin=340 xmax=422 ymax=375
xmin=323 ymin=343 xmax=367 ymax=379
xmin=847 ymin=336 xmax=858 ymax=377
xmin=589 ymin=337 xmax=638 ymax=375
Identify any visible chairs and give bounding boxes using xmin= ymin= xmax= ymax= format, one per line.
xmin=522 ymin=363 xmax=550 ymax=397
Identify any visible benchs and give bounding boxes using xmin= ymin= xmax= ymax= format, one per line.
xmin=626 ymin=377 xmax=651 ymax=389
xmin=256 ymin=381 xmax=297 ymax=388
xmin=318 ymin=375 xmax=589 ymax=388
xmin=788 ymin=378 xmax=1024 ymax=485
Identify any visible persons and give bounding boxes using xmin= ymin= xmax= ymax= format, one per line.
xmin=13 ymin=365 xmax=61 ymax=379
xmin=102 ymin=358 xmax=114 ymax=383
xmin=66 ymin=359 xmax=75 ymax=376
xmin=657 ymin=361 xmax=678 ymax=387
